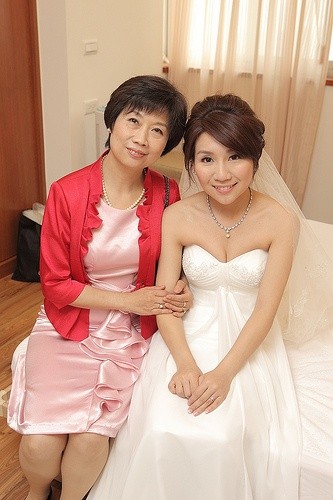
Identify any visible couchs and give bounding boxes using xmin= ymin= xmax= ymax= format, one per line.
xmin=10 ymin=217 xmax=333 ymax=500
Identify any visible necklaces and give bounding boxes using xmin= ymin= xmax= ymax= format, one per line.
xmin=207 ymin=187 xmax=253 ymax=238
xmin=100 ymin=155 xmax=147 ymax=210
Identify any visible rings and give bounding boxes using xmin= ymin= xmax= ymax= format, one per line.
xmin=160 ymin=304 xmax=163 ymax=308
xmin=183 ymin=302 xmax=185 ymax=307
xmin=210 ymin=397 xmax=214 ymax=401
xmin=182 ymin=308 xmax=184 ymax=312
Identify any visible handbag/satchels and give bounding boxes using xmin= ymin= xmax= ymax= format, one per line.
xmin=12 ymin=209 xmax=42 ymax=282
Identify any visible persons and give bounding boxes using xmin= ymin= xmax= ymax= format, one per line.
xmin=87 ymin=93 xmax=302 ymax=500
xmin=6 ymin=75 xmax=194 ymax=500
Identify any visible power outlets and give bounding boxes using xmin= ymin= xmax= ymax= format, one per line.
xmin=84 ymin=98 xmax=99 ymax=116
